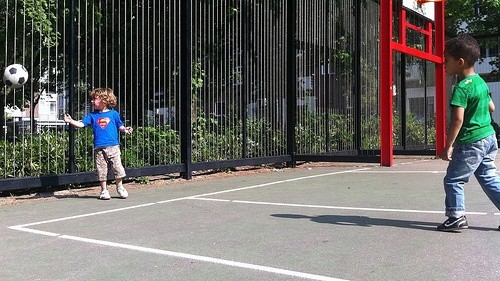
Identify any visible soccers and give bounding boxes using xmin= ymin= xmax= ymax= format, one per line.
xmin=2 ymin=63 xmax=29 ymax=88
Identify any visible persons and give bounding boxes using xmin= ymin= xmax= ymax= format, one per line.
xmin=64 ymin=88 xmax=133 ymax=200
xmin=436 ymin=36 xmax=500 ymax=231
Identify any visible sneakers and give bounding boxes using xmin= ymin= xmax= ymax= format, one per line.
xmin=116 ymin=186 xmax=128 ymax=198
xmin=436 ymin=215 xmax=469 ymax=231
xmin=99 ymin=190 xmax=111 ymax=200
xmin=499 ymin=226 xmax=500 ymax=229
